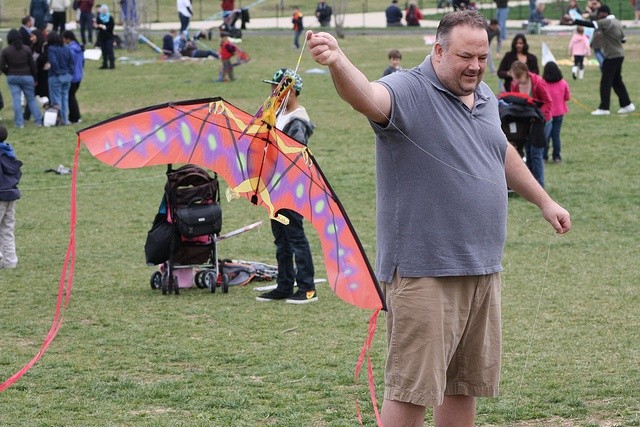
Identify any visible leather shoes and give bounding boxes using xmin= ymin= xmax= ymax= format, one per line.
xmin=99 ymin=65 xmax=108 ymax=68
xmin=109 ymin=64 xmax=115 ymax=68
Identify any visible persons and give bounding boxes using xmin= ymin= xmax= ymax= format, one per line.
xmin=180 ymin=33 xmax=220 ymax=58
xmin=172 ymin=30 xmax=188 ymax=54
xmin=290 ymin=5 xmax=304 ymax=48
xmin=0 ymin=125 xmax=23 ymax=270
xmin=482 ymin=20 xmax=501 ymax=72
xmin=219 ymin=33 xmax=238 ymax=81
xmin=316 ymin=0 xmax=332 ymax=27
xmin=497 ymin=33 xmax=540 ymax=92
xmin=405 ymin=4 xmax=424 ymax=26
xmin=543 ymin=61 xmax=571 ymax=162
xmin=529 ymin=1 xmax=601 ymax=27
xmin=386 ymin=0 xmax=404 ymax=27
xmin=590 ymin=5 xmax=636 ymax=115
xmin=176 ymin=0 xmax=194 ymax=34
xmin=382 ymin=49 xmax=403 ymax=78
xmin=160 ymin=29 xmax=175 ymax=59
xmin=256 ymin=67 xmax=319 ymax=303
xmin=510 ymin=60 xmax=554 ymax=189
xmin=306 ymin=8 xmax=572 ymax=426
xmin=0 ymin=0 xmax=122 ymax=124
xmin=495 ymin=0 xmax=511 ymax=39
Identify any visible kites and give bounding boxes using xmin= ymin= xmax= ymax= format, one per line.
xmin=214 ymin=43 xmax=251 ymax=84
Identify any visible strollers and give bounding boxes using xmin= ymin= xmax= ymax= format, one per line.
xmin=497 ymin=91 xmax=547 ymax=192
xmin=144 ymin=163 xmax=229 ymax=295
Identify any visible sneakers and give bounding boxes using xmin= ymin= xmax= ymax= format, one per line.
xmin=617 ymin=102 xmax=635 ymax=113
xmin=591 ymin=108 xmax=611 ymax=116
xmin=287 ymin=289 xmax=318 ymax=303
xmin=256 ymin=288 xmax=293 ymax=300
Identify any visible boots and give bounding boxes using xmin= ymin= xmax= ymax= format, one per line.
xmin=579 ymin=69 xmax=584 ymax=78
xmin=572 ymin=66 xmax=578 ymax=79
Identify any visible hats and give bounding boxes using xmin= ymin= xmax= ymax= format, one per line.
xmin=263 ymin=68 xmax=303 ymax=92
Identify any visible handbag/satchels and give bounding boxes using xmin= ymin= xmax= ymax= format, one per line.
xmin=169 ymin=182 xmax=222 ymax=235
